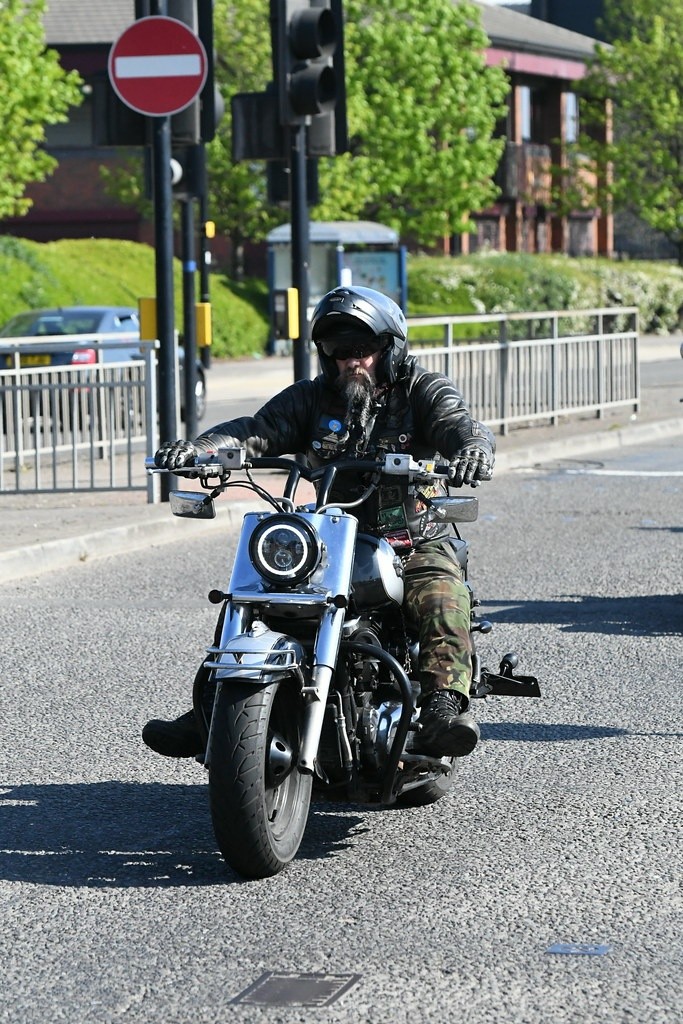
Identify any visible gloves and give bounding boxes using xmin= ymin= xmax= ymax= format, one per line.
xmin=154 ymin=438 xmax=200 ymax=480
xmin=447 ymin=444 xmax=495 ymax=488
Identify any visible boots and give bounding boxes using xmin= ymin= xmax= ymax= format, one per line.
xmin=405 ymin=687 xmax=481 ymax=757
xmin=142 ymin=706 xmax=211 ymax=758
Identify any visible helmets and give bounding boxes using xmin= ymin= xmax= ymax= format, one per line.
xmin=310 ymin=286 xmax=408 ymax=385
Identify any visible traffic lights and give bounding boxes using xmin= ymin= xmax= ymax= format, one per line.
xmin=279 ymin=1 xmax=344 ymax=124
xmin=199 ymin=0 xmax=225 ymax=142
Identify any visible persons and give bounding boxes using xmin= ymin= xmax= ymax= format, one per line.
xmin=142 ymin=286 xmax=497 ymax=757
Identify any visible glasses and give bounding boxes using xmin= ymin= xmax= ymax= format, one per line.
xmin=316 ymin=333 xmax=392 ymax=361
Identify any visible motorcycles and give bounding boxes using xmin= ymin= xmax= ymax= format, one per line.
xmin=139 ymin=421 xmax=540 ymax=883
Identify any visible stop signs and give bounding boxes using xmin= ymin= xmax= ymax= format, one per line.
xmin=107 ymin=14 xmax=209 ymax=118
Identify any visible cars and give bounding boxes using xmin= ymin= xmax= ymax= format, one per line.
xmin=0 ymin=305 xmax=209 ymax=425
